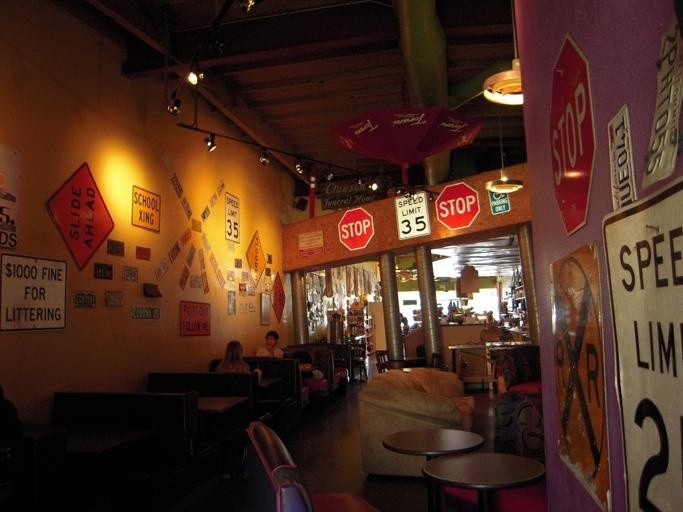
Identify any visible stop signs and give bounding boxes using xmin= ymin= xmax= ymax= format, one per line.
xmin=432 ymin=182 xmax=481 ymax=231
xmin=548 ymin=31 xmax=597 ymax=237
xmin=336 ymin=207 xmax=376 ymax=254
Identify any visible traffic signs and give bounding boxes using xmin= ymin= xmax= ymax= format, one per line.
xmin=392 ymin=191 xmax=431 ymax=241
xmin=226 ymin=192 xmax=240 ymax=242
xmin=599 ymin=177 xmax=682 ymax=512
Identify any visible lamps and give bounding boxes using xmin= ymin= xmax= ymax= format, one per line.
xmin=175 ymin=122 xmax=440 ymax=203
xmin=485 ymin=104 xmax=524 ymax=196
xmin=159 ymin=0 xmax=262 ymax=117
xmin=480 ymin=1 xmax=527 ymax=106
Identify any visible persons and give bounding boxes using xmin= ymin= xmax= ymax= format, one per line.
xmin=399 ymin=313 xmax=410 ymax=335
xmin=256 ymin=331 xmax=285 ymax=359
xmin=219 ymin=341 xmax=250 ymax=375
xmin=1 ymin=386 xmax=25 ymax=484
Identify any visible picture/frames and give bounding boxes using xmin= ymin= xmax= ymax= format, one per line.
xmin=258 ymin=291 xmax=272 ymax=328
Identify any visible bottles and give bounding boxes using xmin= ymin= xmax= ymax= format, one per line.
xmin=447 ymin=300 xmax=458 ymax=315
xmin=510 ymin=269 xmax=524 ymax=299
xmin=350 ymin=324 xmax=357 ymax=335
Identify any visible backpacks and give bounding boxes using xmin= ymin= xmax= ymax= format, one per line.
xmin=292 ymin=350 xmax=313 ymax=379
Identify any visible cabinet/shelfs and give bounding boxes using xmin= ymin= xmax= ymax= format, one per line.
xmin=514 ymin=283 xmax=526 ymax=301
xmin=361 ymin=312 xmax=375 ymax=356
